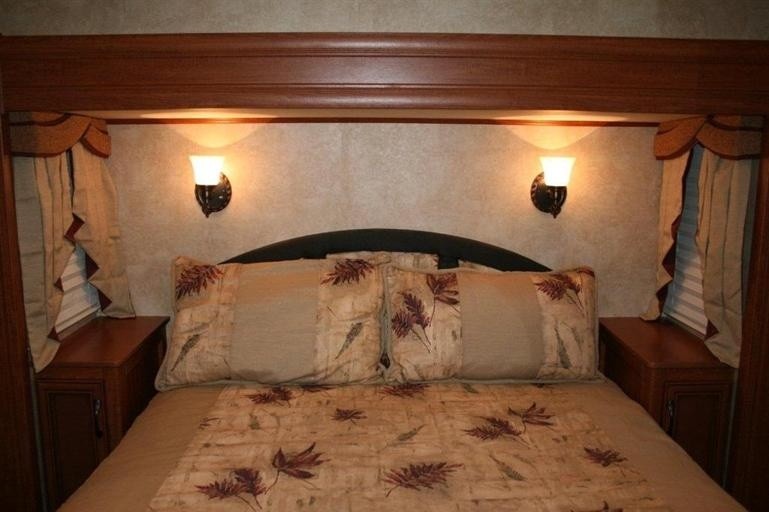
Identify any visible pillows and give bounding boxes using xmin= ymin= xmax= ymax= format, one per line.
xmin=155 ymin=255 xmax=384 ymax=393
xmin=382 ymin=265 xmax=597 ymax=384
xmin=328 ymin=251 xmax=438 ymax=367
xmin=459 ymin=259 xmax=501 ymax=271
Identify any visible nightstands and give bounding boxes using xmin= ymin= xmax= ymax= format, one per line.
xmin=36 ymin=310 xmax=172 ymax=511
xmin=593 ymin=312 xmax=738 ymax=485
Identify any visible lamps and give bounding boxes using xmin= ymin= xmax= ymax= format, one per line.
xmin=529 ymin=153 xmax=575 ymax=219
xmin=189 ymin=155 xmax=232 ymax=220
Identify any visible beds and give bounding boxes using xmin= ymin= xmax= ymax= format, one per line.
xmin=52 ymin=225 xmax=745 ymax=512
